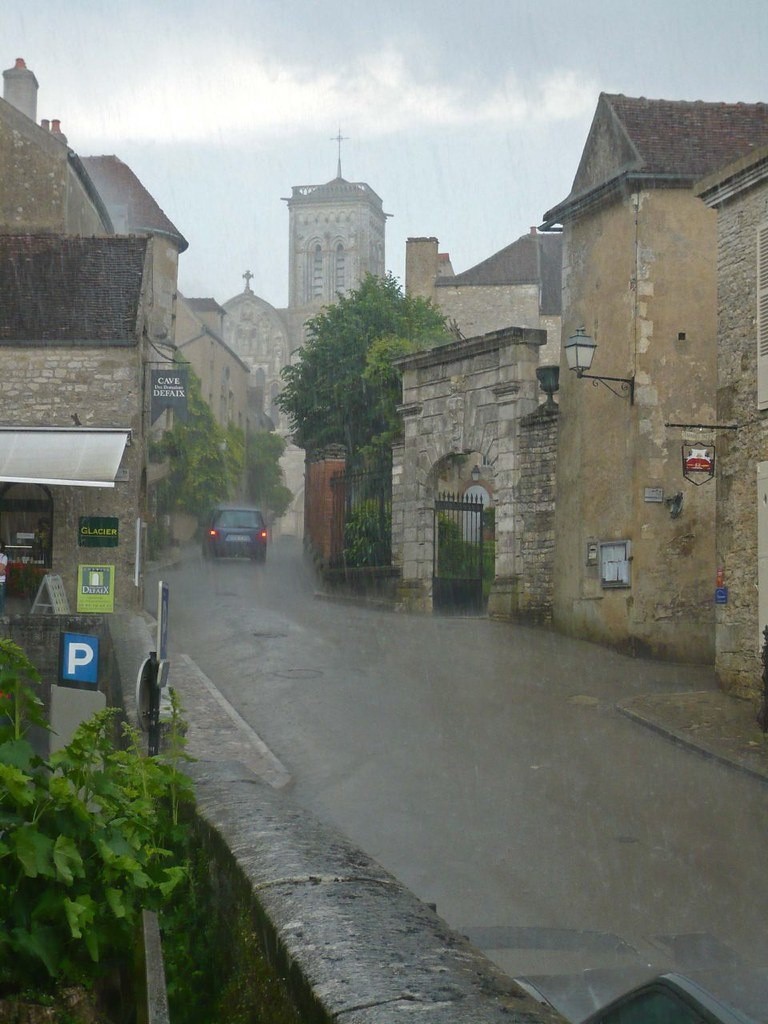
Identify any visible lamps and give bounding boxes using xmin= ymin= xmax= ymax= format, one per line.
xmin=470 ymin=465 xmax=480 ymax=481
xmin=563 ymin=325 xmax=634 ymax=407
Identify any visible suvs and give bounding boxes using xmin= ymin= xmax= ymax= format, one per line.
xmin=201 ymin=505 xmax=268 ymax=564
